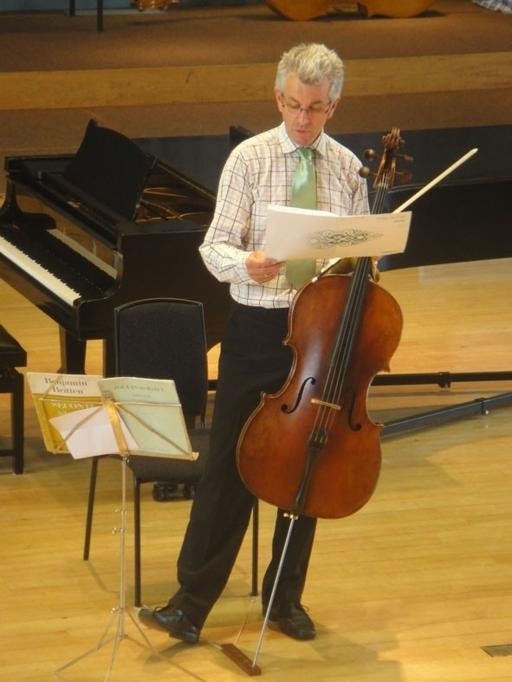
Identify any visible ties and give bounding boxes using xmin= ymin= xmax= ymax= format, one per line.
xmin=285 ymin=148 xmax=318 ymax=291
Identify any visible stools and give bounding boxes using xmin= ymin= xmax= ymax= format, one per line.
xmin=0 ymin=325 xmax=28 ymax=474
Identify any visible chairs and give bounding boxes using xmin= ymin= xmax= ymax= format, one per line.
xmin=84 ymin=294 xmax=259 ymax=608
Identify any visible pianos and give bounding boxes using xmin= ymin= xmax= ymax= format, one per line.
xmin=0 ymin=118 xmax=234 ymax=376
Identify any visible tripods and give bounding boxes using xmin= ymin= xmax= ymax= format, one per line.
xmin=53 ymin=457 xmax=205 ymax=682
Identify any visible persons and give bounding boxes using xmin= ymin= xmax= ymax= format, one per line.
xmin=133 ymin=39 xmax=381 ymax=646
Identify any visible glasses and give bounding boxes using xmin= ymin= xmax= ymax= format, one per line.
xmin=278 ymin=94 xmax=332 ymax=117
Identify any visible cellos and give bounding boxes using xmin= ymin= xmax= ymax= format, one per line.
xmin=234 ymin=126 xmax=414 ymax=518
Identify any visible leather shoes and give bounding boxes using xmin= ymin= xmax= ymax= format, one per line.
xmin=139 ymin=607 xmax=200 ymax=642
xmin=263 ymin=600 xmax=314 ymax=640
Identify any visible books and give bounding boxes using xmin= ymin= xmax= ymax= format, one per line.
xmin=260 ymin=197 xmax=414 ymax=266
xmin=99 ymin=376 xmax=202 ymax=465
xmin=26 ymin=366 xmax=126 ymax=460
